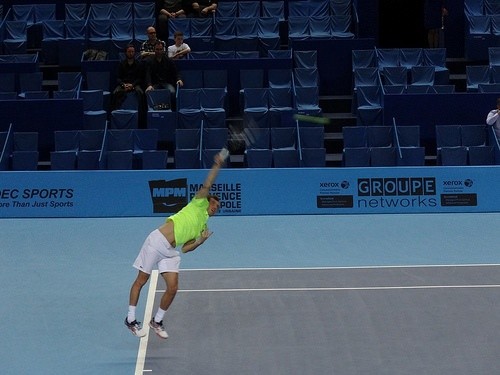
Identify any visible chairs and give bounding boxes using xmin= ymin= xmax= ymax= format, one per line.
xmin=0 ymin=0 xmax=500 ymax=171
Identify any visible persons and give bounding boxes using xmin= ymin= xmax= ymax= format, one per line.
xmin=485 ymin=97 xmax=499 ymax=140
xmin=154 ymin=0 xmax=217 ymax=36
xmin=124 ymin=147 xmax=228 ymax=339
xmin=112 ymin=26 xmax=191 ymax=111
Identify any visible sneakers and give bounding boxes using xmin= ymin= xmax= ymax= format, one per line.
xmin=149 ymin=317 xmax=169 ymax=339
xmin=124 ymin=317 xmax=146 ymax=337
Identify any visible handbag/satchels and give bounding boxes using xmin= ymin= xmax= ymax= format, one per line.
xmin=80 ymin=46 xmax=108 ymax=61
